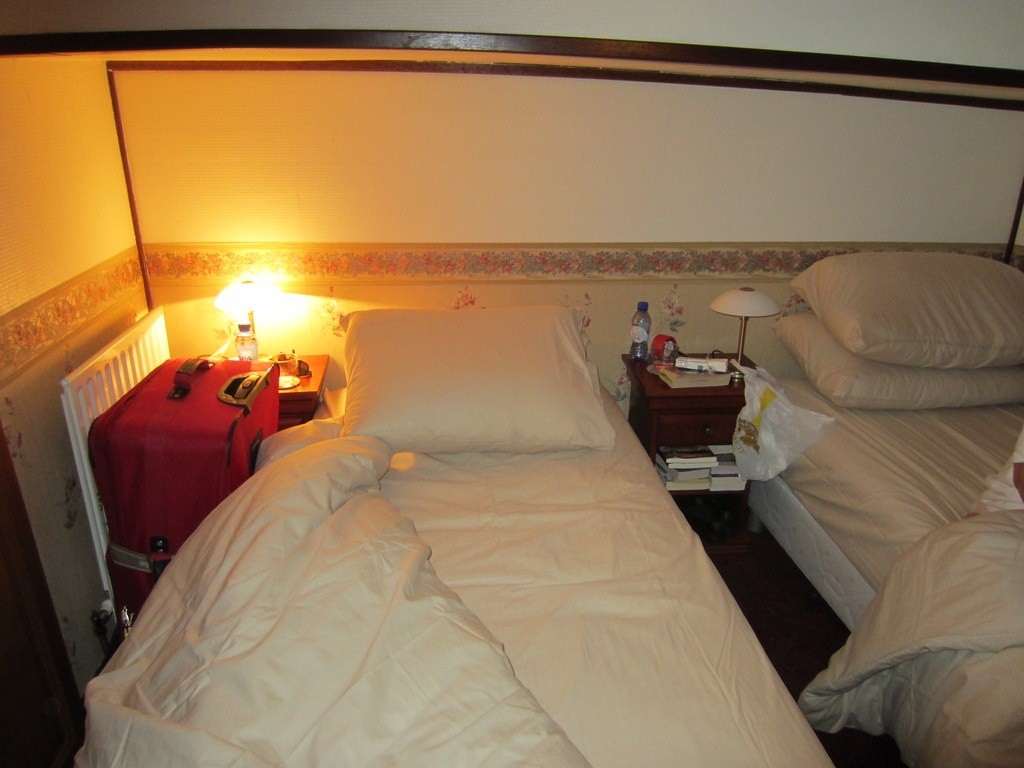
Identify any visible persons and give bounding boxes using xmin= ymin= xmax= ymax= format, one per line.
xmin=962 ymin=425 xmax=1024 ymax=518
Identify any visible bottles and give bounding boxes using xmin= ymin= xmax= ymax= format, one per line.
xmin=629 ymin=302 xmax=650 ymax=361
xmin=235 ymin=324 xmax=258 ymax=362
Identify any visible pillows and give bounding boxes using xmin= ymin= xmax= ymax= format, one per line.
xmin=339 ymin=305 xmax=616 ymax=452
xmin=772 ymin=314 xmax=1024 ymax=408
xmin=787 ymin=251 xmax=1024 ymax=368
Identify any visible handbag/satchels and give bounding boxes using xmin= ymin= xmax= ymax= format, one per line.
xmin=728 ymin=356 xmax=838 ymax=483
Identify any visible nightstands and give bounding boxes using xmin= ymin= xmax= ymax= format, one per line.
xmin=622 ymin=352 xmax=761 ymax=552
xmin=276 ymin=353 xmax=330 ymax=429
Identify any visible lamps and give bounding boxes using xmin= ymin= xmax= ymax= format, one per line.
xmin=215 ymin=276 xmax=277 ymax=360
xmin=709 ymin=287 xmax=782 ymax=377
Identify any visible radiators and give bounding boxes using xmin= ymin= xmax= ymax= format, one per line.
xmin=59 ymin=303 xmax=171 ymax=611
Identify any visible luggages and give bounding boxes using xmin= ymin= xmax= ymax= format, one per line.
xmin=84 ymin=355 xmax=279 ymax=643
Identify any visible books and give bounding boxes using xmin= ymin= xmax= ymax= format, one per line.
xmin=656 ymin=367 xmax=730 ymax=388
xmin=658 ymin=445 xmax=719 ymax=469
xmin=705 ymin=445 xmax=746 ymax=491
xmin=655 ymin=453 xmax=710 ymax=490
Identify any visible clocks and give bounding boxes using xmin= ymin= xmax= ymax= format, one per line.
xmin=652 ymin=334 xmax=679 ymax=364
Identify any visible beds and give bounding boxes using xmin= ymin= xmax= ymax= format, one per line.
xmin=749 ymin=359 xmax=1024 ymax=767
xmin=74 ymin=387 xmax=831 ymax=768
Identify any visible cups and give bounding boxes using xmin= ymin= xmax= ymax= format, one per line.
xmin=657 ymin=342 xmax=679 ymax=367
